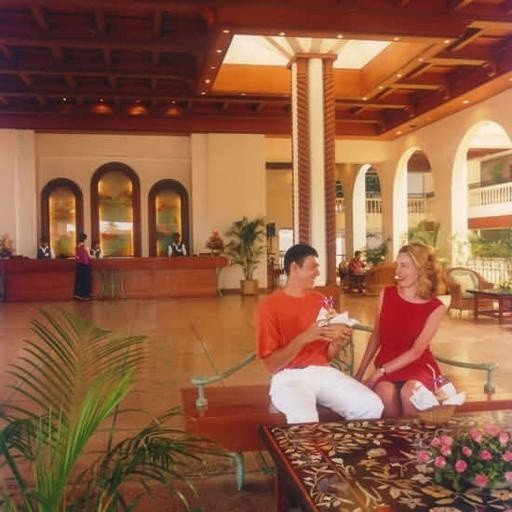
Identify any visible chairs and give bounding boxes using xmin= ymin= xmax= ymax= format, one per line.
xmin=339 ymin=258 xmax=399 ymax=300
xmin=445 ymin=267 xmax=495 ymax=321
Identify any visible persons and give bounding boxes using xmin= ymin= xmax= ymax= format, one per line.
xmin=339 ymin=254 xmax=350 ymax=289
xmin=91 ymin=240 xmax=103 ymax=259
xmin=36 ymin=236 xmax=51 ymax=259
xmin=73 ymin=232 xmax=92 ymax=301
xmin=167 ymin=231 xmax=187 ymax=257
xmin=256 ymin=242 xmax=384 ymax=424
xmin=348 ymin=250 xmax=366 ymax=293
xmin=352 ymin=240 xmax=449 ymax=418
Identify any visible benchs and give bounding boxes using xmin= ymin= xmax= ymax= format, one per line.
xmin=180 ymin=323 xmax=499 ymax=492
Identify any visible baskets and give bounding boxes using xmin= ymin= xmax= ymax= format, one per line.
xmin=417 ymin=403 xmax=458 ymax=425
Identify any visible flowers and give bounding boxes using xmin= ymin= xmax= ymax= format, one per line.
xmin=417 ymin=423 xmax=512 ymax=494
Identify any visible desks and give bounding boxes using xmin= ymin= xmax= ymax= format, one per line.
xmin=466 ymin=287 xmax=512 ymax=324
xmin=258 ymin=399 xmax=512 ymax=512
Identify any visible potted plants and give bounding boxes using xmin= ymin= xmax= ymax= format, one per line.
xmin=224 ymin=216 xmax=268 ymax=293
